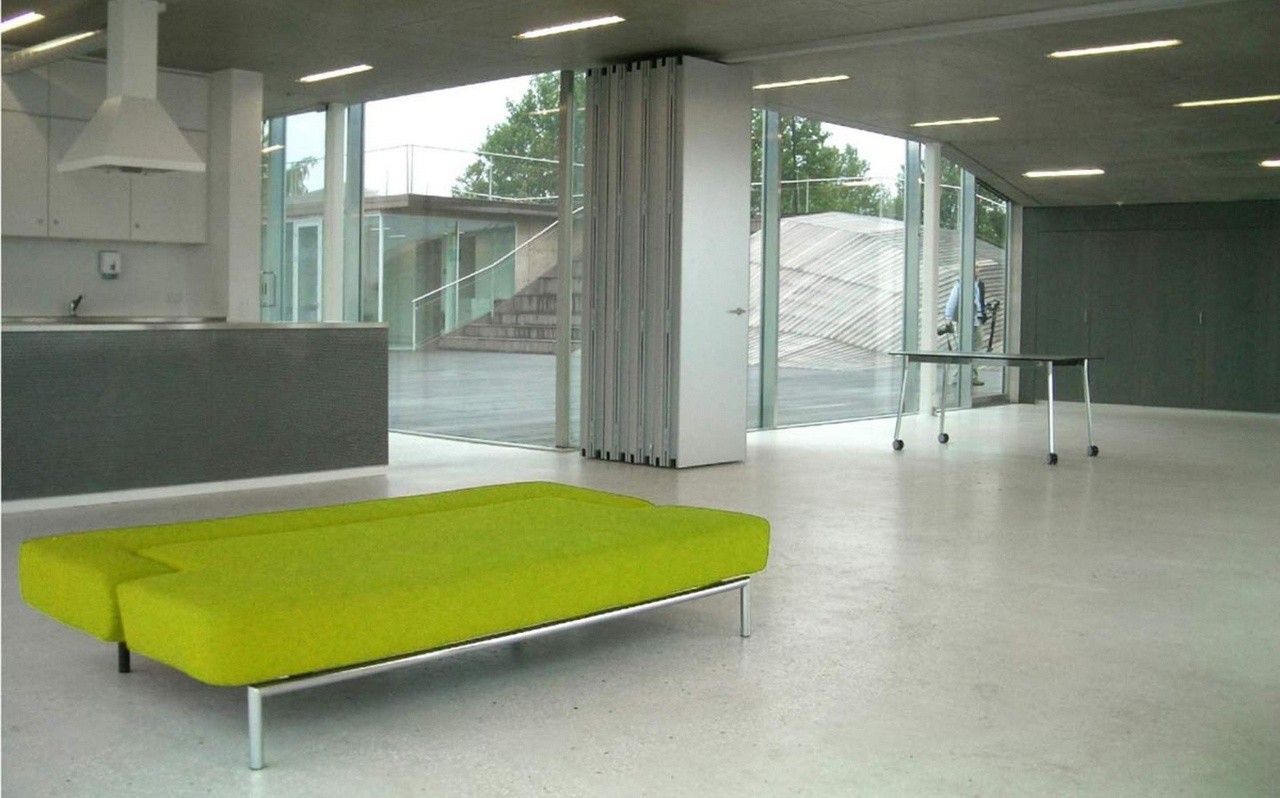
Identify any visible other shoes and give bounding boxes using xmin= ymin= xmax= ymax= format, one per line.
xmin=973 ymin=377 xmax=983 ymax=386
xmin=953 ymin=377 xmax=958 ymax=384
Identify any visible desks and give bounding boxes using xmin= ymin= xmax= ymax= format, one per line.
xmin=888 ymin=352 xmax=1107 ymax=466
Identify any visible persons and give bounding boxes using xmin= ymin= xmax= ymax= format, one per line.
xmin=948 ymin=263 xmax=985 ymax=385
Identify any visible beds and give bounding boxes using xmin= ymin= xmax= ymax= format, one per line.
xmin=19 ymin=482 xmax=767 ymax=770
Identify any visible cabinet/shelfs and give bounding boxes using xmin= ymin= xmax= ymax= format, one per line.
xmin=2 ymin=49 xmax=211 ymax=242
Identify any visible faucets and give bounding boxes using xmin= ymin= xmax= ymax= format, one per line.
xmin=67 ymin=294 xmax=82 ymax=316
